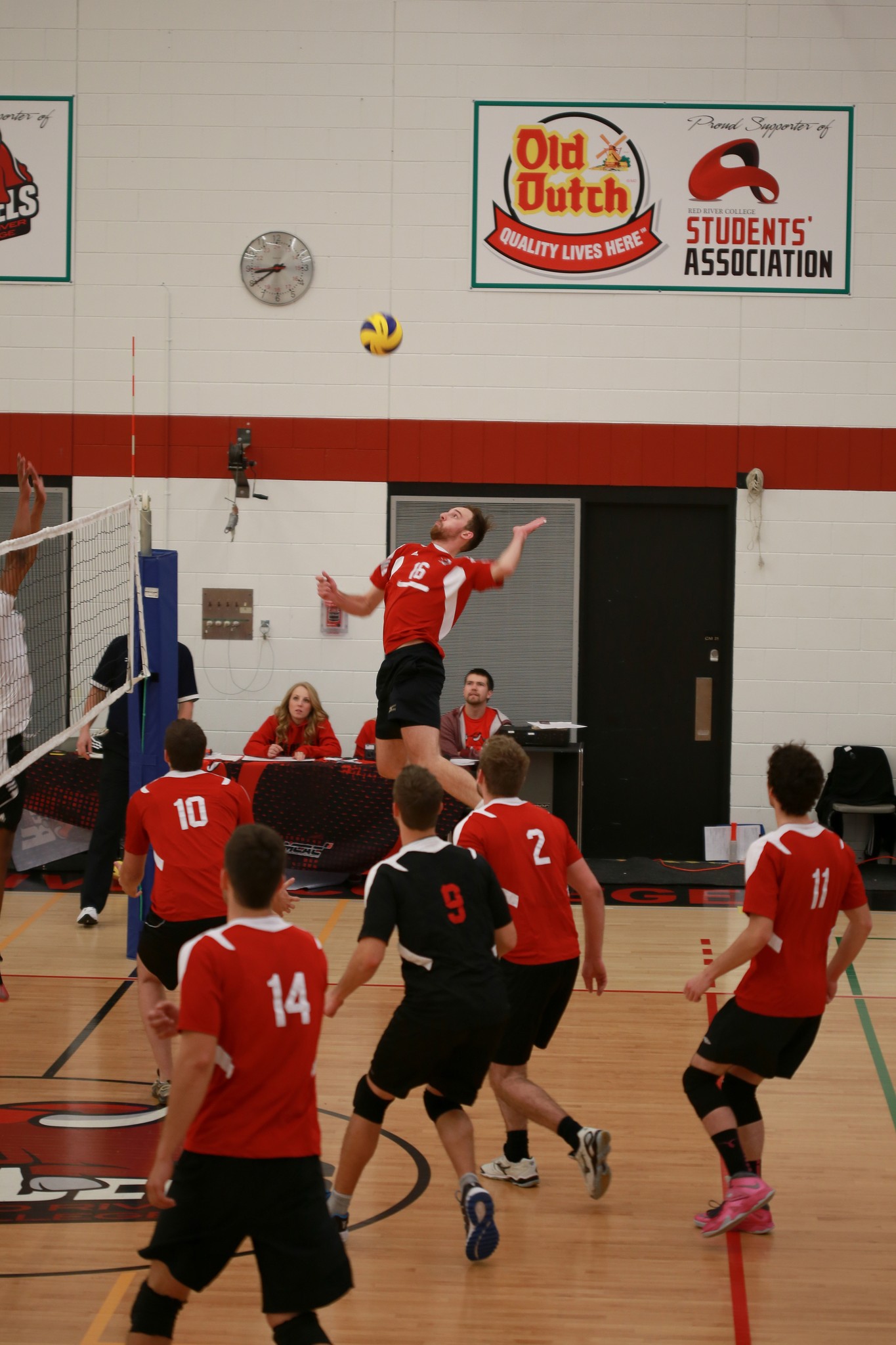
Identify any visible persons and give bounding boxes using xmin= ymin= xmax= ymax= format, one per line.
xmin=120 ymin=719 xmax=300 ymax=1103
xmin=683 ymin=738 xmax=872 ymax=1239
xmin=243 ymin=681 xmax=341 ymax=761
xmin=125 ymin=826 xmax=354 ymax=1345
xmin=325 ymin=766 xmax=517 ymax=1262
xmin=0 ymin=454 xmax=46 ymax=1001
xmin=353 ymin=698 xmax=376 ymax=761
xmin=441 ymin=667 xmax=513 ymax=761
xmin=77 ymin=631 xmax=199 ymax=926
xmin=317 ymin=507 xmax=546 ymax=812
xmin=454 ymin=733 xmax=611 ymax=1199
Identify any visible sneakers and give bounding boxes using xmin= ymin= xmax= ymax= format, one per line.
xmin=693 ymin=1171 xmax=775 ymax=1238
xmin=150 ymin=1067 xmax=172 ymax=1104
xmin=566 ymin=1126 xmax=612 ymax=1200
xmin=479 ymin=1143 xmax=540 ymax=1187
xmin=459 ymin=1181 xmax=500 ymax=1261
xmin=76 ymin=907 xmax=99 ymax=925
xmin=326 ymin=1190 xmax=349 ymax=1241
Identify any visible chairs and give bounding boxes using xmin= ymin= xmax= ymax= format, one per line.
xmin=816 ymin=746 xmax=896 ymax=864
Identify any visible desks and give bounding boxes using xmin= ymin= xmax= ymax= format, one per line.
xmin=514 ymin=743 xmax=585 ymax=859
xmin=9 ymin=748 xmax=481 ymax=893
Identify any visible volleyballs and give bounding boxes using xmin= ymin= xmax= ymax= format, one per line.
xmin=360 ymin=314 xmax=402 ymax=355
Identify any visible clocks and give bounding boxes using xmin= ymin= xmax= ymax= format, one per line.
xmin=240 ymin=230 xmax=314 ymax=307
